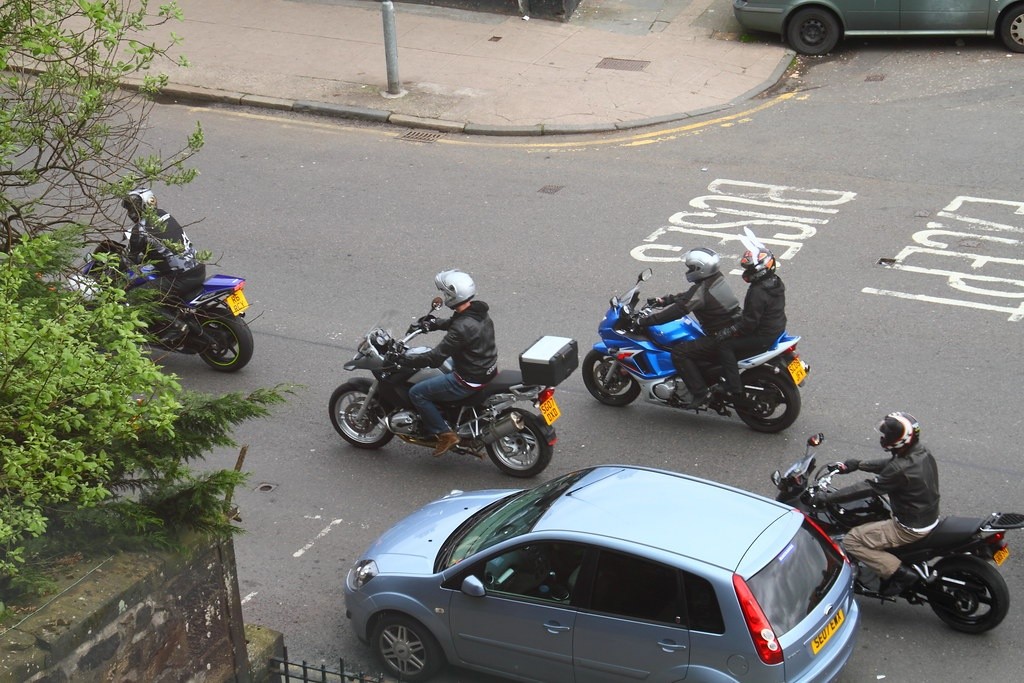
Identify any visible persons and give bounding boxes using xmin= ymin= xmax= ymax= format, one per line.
xmin=829 ymin=412 xmax=941 ymax=597
xmin=94 ymin=187 xmax=206 ymax=343
xmin=627 ymin=247 xmax=786 ymax=410
xmin=384 ymin=270 xmax=498 ymax=457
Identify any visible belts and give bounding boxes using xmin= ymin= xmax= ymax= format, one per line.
xmin=453 ymin=371 xmax=498 ymax=391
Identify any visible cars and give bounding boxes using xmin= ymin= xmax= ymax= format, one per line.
xmin=732 ymin=0 xmax=1024 ymax=59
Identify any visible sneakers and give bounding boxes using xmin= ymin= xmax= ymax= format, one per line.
xmin=433 ymin=432 xmax=460 ymax=456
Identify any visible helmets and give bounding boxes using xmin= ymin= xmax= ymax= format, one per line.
xmin=685 ymin=248 xmax=720 ymax=282
xmin=741 ymin=247 xmax=776 ymax=283
xmin=122 ymin=189 xmax=156 ymax=217
xmin=879 ymin=412 xmax=920 ymax=453
xmin=435 ymin=269 xmax=476 ymax=309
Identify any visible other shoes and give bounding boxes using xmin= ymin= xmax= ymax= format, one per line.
xmin=883 ymin=568 xmax=919 ymax=596
xmin=170 ymin=322 xmax=200 ymax=344
xmin=727 ymin=393 xmax=746 ymax=402
xmin=686 ymin=388 xmax=712 ymax=408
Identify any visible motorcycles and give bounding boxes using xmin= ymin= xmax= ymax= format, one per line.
xmin=61 ymin=225 xmax=257 ymax=373
xmin=770 ymin=433 xmax=1024 ymax=635
xmin=327 ymin=296 xmax=580 ymax=478
xmin=581 ymin=267 xmax=810 ymax=435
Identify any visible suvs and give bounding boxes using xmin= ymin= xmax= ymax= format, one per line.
xmin=342 ymin=463 xmax=862 ymax=682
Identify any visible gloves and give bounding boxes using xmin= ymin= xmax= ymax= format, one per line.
xmin=647 ymin=297 xmax=663 ymax=307
xmin=382 ymin=352 xmax=406 ymax=373
xmin=812 ymin=491 xmax=830 ymax=507
xmin=714 ymin=325 xmax=740 ymax=343
xmin=625 ymin=317 xmax=639 ymax=331
xmin=418 ymin=315 xmax=436 ymax=332
xmin=839 ymin=459 xmax=859 ymax=474
